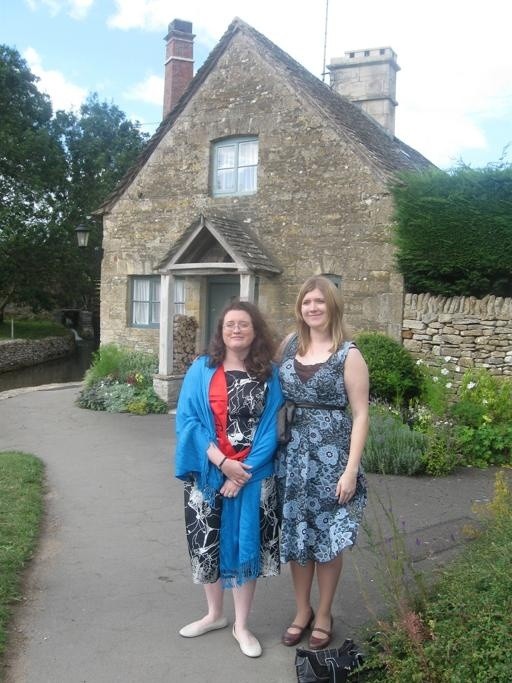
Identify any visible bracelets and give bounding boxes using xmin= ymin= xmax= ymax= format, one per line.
xmin=217 ymin=455 xmax=227 ymax=468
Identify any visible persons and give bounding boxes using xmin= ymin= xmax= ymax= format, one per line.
xmin=173 ymin=298 xmax=286 ymax=657
xmin=270 ymin=272 xmax=371 ymax=651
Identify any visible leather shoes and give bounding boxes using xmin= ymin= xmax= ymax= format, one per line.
xmin=178 ymin=614 xmax=228 ymax=637
xmin=309 ymin=615 xmax=333 ymax=650
xmin=282 ymin=607 xmax=314 ymax=646
xmin=232 ymin=624 xmax=261 ymax=658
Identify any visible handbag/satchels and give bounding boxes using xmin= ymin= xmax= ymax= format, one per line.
xmin=295 ymin=639 xmax=365 ymax=683
